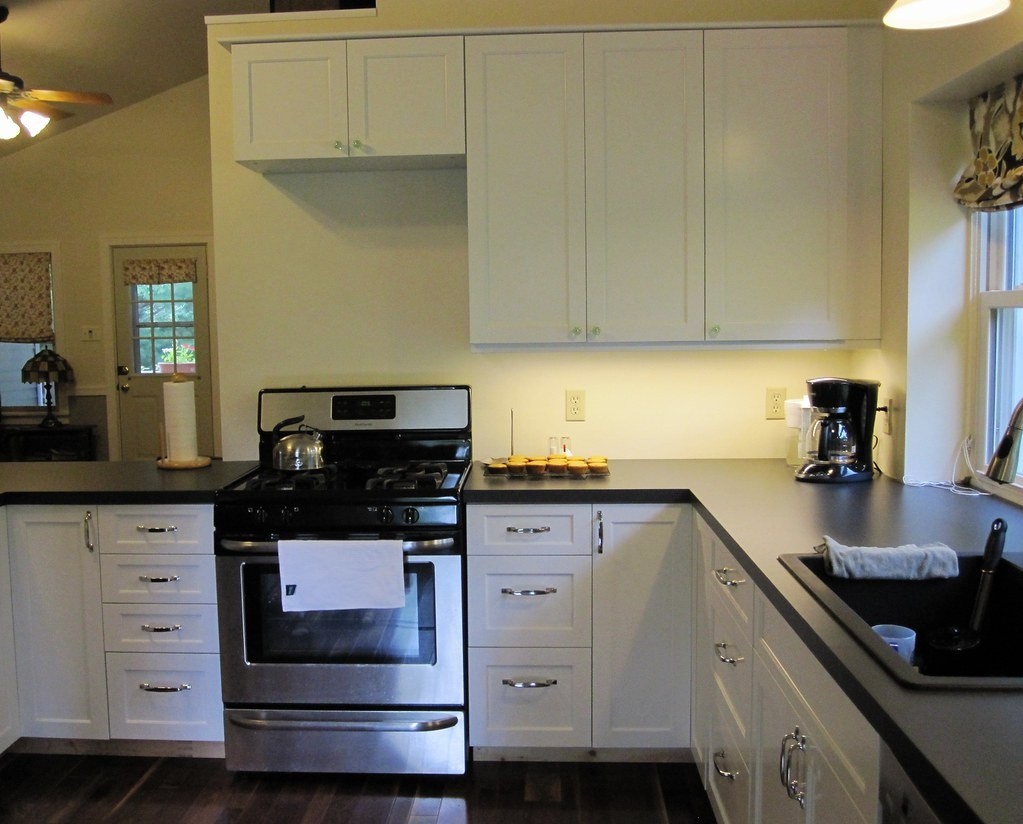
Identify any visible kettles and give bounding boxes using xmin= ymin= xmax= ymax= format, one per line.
xmin=271 ymin=415 xmax=328 ymax=472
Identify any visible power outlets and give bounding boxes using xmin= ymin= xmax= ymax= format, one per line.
xmin=765 ymin=386 xmax=786 ymax=420
xmin=565 ymin=388 xmax=586 ymax=421
xmin=882 ymin=397 xmax=893 ymax=435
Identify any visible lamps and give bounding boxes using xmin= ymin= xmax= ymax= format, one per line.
xmin=21 ymin=343 xmax=76 ymax=428
xmin=883 ymin=0 xmax=1010 ymax=30
xmin=0 ymin=102 xmax=53 ymax=143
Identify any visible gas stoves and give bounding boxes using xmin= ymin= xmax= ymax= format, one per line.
xmin=210 ymin=384 xmax=473 ymax=539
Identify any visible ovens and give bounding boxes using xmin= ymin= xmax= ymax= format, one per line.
xmin=213 ymin=536 xmax=469 ymax=784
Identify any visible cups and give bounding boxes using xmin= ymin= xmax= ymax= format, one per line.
xmin=872 ymin=624 xmax=916 ymax=666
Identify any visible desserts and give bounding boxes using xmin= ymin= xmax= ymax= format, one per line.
xmin=487 ymin=455 xmax=608 ymax=475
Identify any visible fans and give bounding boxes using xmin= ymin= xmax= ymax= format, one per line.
xmin=0 ymin=5 xmax=115 ymax=122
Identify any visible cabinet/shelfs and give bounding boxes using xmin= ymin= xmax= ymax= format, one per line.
xmin=465 ymin=504 xmax=889 ymax=824
xmin=461 ymin=29 xmax=704 ymax=352
xmin=228 ymin=36 xmax=466 ymax=173
xmin=705 ymin=20 xmax=888 ymax=353
xmin=0 ymin=494 xmax=225 ymax=767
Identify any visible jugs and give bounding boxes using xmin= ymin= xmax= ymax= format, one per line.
xmin=805 ymin=416 xmax=857 ymax=464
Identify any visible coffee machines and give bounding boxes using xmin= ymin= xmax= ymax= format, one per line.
xmin=792 ymin=376 xmax=883 ymax=484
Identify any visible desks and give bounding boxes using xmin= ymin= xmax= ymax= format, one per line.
xmin=0 ymin=423 xmax=99 ymax=462
